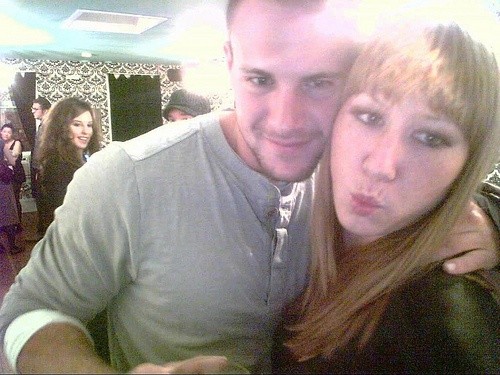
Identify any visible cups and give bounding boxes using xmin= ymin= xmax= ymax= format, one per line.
xmin=171 ymin=358 xmax=249 ymax=375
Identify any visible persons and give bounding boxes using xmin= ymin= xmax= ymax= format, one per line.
xmin=0 ymin=123 xmax=27 ymax=234
xmin=270 ymin=18 xmax=500 ymax=375
xmin=1 ymin=0 xmax=500 ymax=375
xmin=0 ymin=136 xmax=25 ymax=256
xmin=33 ymin=96 xmax=111 ymax=366
xmin=163 ymin=88 xmax=212 ymax=124
xmin=24 ymin=97 xmax=51 ymax=243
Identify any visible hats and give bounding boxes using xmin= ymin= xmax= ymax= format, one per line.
xmin=163 ymin=89 xmax=211 ymax=121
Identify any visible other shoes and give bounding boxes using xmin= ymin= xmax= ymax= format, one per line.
xmin=10 ymin=245 xmax=25 ymax=254
xmin=25 ymin=233 xmax=43 ymax=242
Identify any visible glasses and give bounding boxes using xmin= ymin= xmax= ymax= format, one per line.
xmin=30 ymin=107 xmax=43 ymax=111
